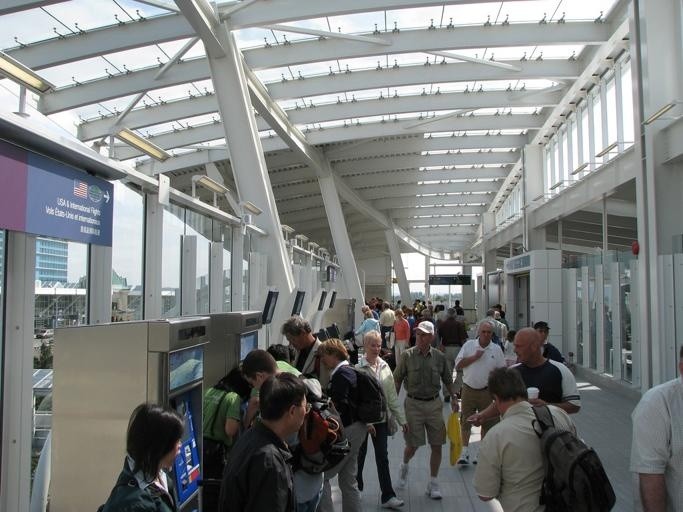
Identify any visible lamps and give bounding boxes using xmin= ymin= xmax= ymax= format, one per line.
xmin=0 ymin=51 xmax=56 ymax=97
xmin=114 ymin=126 xmax=171 ymax=164
xmin=243 ymin=200 xmax=263 ymax=216
xmin=195 ymin=174 xmax=229 ymax=196
xmin=279 ymin=223 xmax=338 ymax=262
xmin=477 ymin=100 xmax=683 ymax=239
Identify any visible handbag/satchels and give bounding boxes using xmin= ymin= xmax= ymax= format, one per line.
xmin=531 ymin=419 xmax=616 ymax=512
xmin=203 ymin=438 xmax=226 ymax=479
xmin=295 ymin=393 xmax=351 ymax=474
xmin=385 ymin=331 xmax=396 ymax=350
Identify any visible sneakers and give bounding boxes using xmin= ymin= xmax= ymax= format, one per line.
xmin=382 ymin=496 xmax=405 ymax=508
xmin=445 ymin=394 xmax=450 ymax=402
xmin=473 ymin=448 xmax=481 ymax=463
xmin=457 ymin=449 xmax=470 ymax=465
xmin=425 ymin=481 xmax=442 ymax=498
xmin=397 ymin=464 xmax=409 ymax=487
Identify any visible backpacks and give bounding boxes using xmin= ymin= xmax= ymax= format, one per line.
xmin=337 ymin=365 xmax=387 ymax=424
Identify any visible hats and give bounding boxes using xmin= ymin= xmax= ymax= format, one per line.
xmin=534 ymin=321 xmax=551 ymax=330
xmin=412 ymin=320 xmax=435 ymax=335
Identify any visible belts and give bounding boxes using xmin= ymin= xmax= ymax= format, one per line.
xmin=406 ymin=392 xmax=439 ymax=402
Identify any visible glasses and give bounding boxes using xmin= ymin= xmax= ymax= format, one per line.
xmin=289 ymin=402 xmax=313 ymax=415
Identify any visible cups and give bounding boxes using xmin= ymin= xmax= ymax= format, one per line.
xmin=527 ymin=389 xmax=539 ymax=400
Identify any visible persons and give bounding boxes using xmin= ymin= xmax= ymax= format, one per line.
xmin=631 ymin=348 xmax=683 ymax=512
xmin=203 ymin=297 xmax=582 ymax=511
xmin=102 ymin=404 xmax=184 ymax=512
